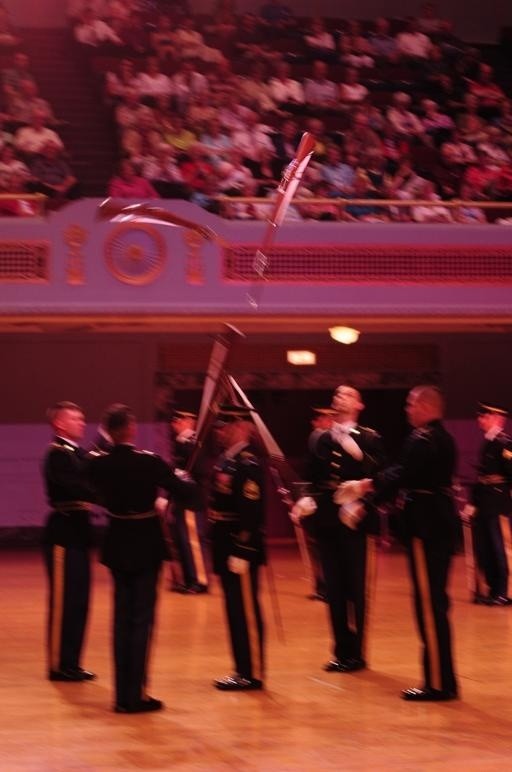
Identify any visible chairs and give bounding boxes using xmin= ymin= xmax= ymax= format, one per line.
xmin=0 ymin=0 xmax=511 ymax=222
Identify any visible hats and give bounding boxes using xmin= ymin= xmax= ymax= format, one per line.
xmin=472 ymin=398 xmax=510 ymax=419
xmin=208 ymin=402 xmax=259 ymax=429
xmin=167 ymin=407 xmax=200 ymax=421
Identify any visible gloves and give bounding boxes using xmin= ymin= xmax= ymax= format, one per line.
xmin=289 ymin=424 xmax=372 ymax=532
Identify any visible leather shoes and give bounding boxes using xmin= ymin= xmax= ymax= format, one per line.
xmin=323 ymin=654 xmax=367 ymax=675
xmin=47 ymin=669 xmax=98 ymax=683
xmin=210 ymin=673 xmax=267 ymax=693
xmin=169 ymin=581 xmax=210 ymax=596
xmin=114 ymin=699 xmax=165 ymax=716
xmin=402 ymin=681 xmax=463 ymax=706
xmin=472 ymin=590 xmax=511 ymax=607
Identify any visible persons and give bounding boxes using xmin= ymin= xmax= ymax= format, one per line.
xmin=40 ymin=399 xmax=113 ymax=678
xmin=462 ymin=402 xmax=510 ymax=609
xmin=332 ymin=387 xmax=461 ymax=702
xmin=199 ymin=397 xmax=270 ymax=690
xmin=302 ymin=406 xmax=332 ymax=606
xmin=291 ymin=382 xmax=385 ymax=671
xmin=166 ymin=407 xmax=211 ymax=594
xmin=84 ymin=405 xmax=203 ymax=713
xmin=66 ymin=2 xmax=511 ymax=223
xmin=0 ymin=4 xmax=77 ymax=206
